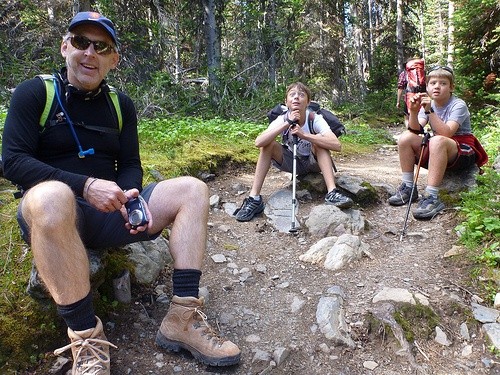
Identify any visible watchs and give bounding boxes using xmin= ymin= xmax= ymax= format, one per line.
xmin=425 ymin=108 xmax=434 ymax=114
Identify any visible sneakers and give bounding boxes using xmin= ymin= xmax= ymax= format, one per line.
xmin=413 ymin=194 xmax=446 ymax=220
xmin=53 ymin=314 xmax=118 ymax=375
xmin=235 ymin=195 xmax=265 ymax=222
xmin=324 ymin=188 xmax=354 ymax=209
xmin=156 ymin=295 xmax=241 ymax=366
xmin=388 ymin=183 xmax=418 ymax=207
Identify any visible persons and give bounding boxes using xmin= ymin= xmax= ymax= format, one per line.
xmin=396 ymin=58 xmax=426 ymax=130
xmin=236 ymin=82 xmax=354 ymax=222
xmin=2 ymin=11 xmax=240 ymax=375
xmin=387 ymin=65 xmax=488 ymax=218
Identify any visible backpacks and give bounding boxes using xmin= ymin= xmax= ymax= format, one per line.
xmin=406 ymin=59 xmax=426 ymax=92
xmin=267 ymin=102 xmax=346 ymax=173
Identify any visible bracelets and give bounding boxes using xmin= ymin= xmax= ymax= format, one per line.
xmin=86 ymin=178 xmax=98 ymax=207
xmin=286 ymin=118 xmax=293 ymax=125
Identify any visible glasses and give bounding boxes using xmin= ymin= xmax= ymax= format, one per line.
xmin=427 ymin=65 xmax=454 ymax=75
xmin=63 ymin=36 xmax=117 ymax=56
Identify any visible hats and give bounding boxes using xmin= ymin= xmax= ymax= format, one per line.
xmin=67 ymin=11 xmax=118 ymax=47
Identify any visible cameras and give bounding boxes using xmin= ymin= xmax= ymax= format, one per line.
xmin=125 ymin=196 xmax=149 ymax=229
